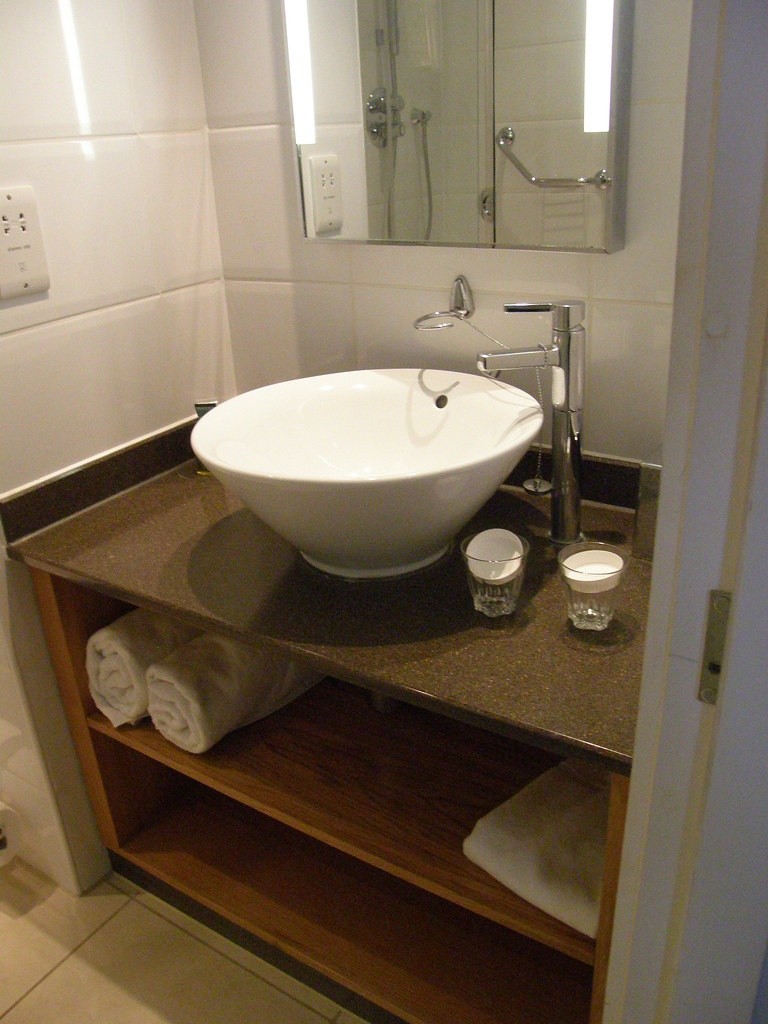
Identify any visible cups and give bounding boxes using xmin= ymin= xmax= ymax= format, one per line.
xmin=559 ymin=541 xmax=630 ymax=632
xmin=460 ymin=533 xmax=530 ymax=619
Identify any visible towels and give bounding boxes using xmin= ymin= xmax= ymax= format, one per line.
xmin=461 ymin=756 xmax=609 ymax=941
xmin=145 ymin=631 xmax=329 ymax=755
xmin=84 ymin=606 xmax=205 ymax=729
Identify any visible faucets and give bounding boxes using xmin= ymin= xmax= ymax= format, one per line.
xmin=475 ymin=299 xmax=587 ymax=544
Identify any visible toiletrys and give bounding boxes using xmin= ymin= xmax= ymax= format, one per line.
xmin=193 ymin=397 xmax=218 ymax=417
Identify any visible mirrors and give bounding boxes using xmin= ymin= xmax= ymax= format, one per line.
xmin=278 ymin=0 xmax=634 ymax=259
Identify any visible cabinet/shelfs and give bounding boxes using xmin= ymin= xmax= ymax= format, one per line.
xmin=7 ymin=457 xmax=650 ymax=1024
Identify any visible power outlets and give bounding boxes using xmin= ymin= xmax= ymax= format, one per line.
xmin=310 ymin=154 xmax=343 ymax=233
xmin=0 ymin=186 xmax=51 ymax=295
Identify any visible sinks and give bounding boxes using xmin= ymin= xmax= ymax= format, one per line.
xmin=190 ymin=367 xmax=545 ymax=579
